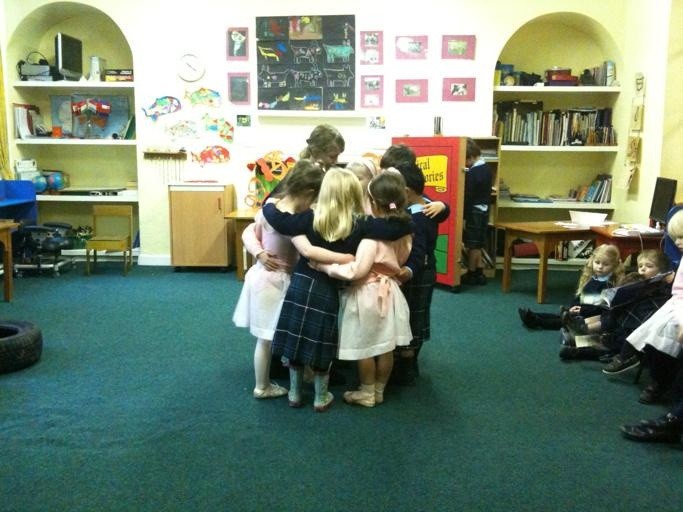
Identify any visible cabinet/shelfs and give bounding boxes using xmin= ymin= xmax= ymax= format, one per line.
xmin=493 ymin=84 xmax=622 ymax=272
xmin=391 ymin=134 xmax=504 ymax=287
xmin=167 ymin=183 xmax=235 ymax=272
xmin=12 ymin=80 xmax=138 ymax=256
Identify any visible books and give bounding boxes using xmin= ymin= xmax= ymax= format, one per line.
xmin=499 ymin=174 xmax=612 ymax=203
xmin=462 ymin=243 xmax=494 ymax=269
xmin=18 ymin=32 xmax=134 ymax=81
xmin=480 ymin=99 xmax=616 ymax=160
xmin=12 ymin=103 xmax=41 ymax=140
xmin=15 ymin=159 xmax=41 ymax=181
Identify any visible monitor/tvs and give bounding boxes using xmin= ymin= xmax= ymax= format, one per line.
xmin=55 ymin=32 xmax=82 ymax=81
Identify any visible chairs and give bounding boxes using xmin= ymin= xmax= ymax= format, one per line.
xmin=84 ymin=204 xmax=133 ymax=277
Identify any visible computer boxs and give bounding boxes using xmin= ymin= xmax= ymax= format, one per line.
xmin=20 ymin=64 xmax=55 ymax=76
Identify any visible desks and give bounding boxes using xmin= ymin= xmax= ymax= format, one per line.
xmin=0 ymin=218 xmax=21 ymax=303
xmin=589 ymin=223 xmax=664 ymax=264
xmin=225 ymin=208 xmax=258 ymax=278
xmin=492 ymin=219 xmax=620 ymax=305
xmin=0 ymin=179 xmax=37 ymax=226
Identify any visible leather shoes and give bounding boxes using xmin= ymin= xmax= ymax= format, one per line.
xmin=619 ymin=382 xmax=683 ymax=444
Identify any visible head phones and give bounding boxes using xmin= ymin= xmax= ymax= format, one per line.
xmin=26 ymin=51 xmax=49 ymax=65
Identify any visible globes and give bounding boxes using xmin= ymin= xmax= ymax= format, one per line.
xmin=32 ymin=175 xmax=50 ymax=194
xmin=48 ymin=172 xmax=65 ymax=195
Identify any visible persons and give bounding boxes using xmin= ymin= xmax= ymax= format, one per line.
xmin=518 ymin=208 xmax=683 ymax=444
xmin=463 ymin=137 xmax=492 ymax=286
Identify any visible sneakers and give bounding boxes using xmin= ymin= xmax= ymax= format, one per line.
xmin=559 ymin=327 xmax=574 ymax=346
xmin=557 ymin=344 xmax=579 ymax=361
xmin=252 ymin=383 xmax=305 ymax=408
xmin=602 ymin=353 xmax=642 ymax=376
xmin=559 ymin=304 xmax=578 ymax=334
xmin=342 ymin=389 xmax=376 ymax=409
xmin=461 ymin=272 xmax=487 ymax=286
xmin=312 ymin=390 xmax=336 ymax=412
